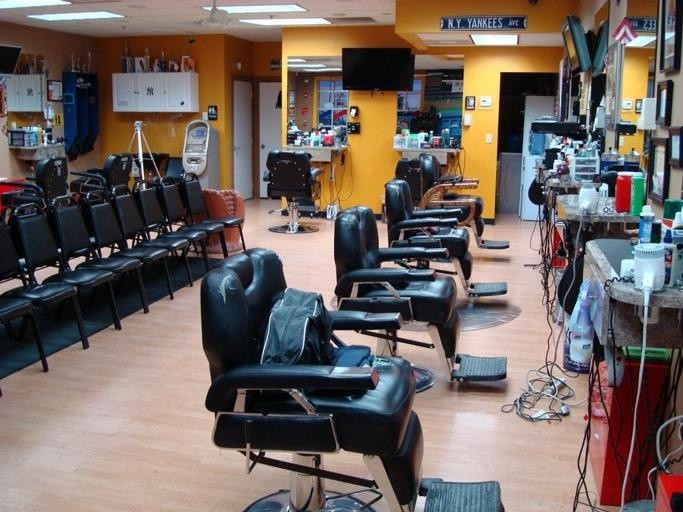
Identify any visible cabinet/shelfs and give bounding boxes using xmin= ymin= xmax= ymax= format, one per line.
xmin=112 ymin=72 xmax=199 ymax=113
xmin=585 ymin=341 xmax=670 ymax=506
xmin=62 ymin=70 xmax=100 ymax=157
xmin=522 ymin=95 xmax=555 ymax=158
xmin=9 ymin=141 xmax=68 ymax=176
xmin=580 ymin=236 xmax=682 ymax=388
xmin=532 ymin=162 xmax=655 ymax=252
xmin=7 ymin=73 xmax=48 ymax=112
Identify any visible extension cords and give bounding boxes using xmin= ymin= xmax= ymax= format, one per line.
xmin=545 ymin=378 xmax=566 ymax=394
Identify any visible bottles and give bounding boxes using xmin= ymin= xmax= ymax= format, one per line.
xmin=638 ymin=204 xmax=655 ymax=243
xmin=661 ymin=230 xmax=675 ymax=290
xmin=613 ymin=171 xmax=645 ymax=216
xmin=562 ymin=279 xmax=594 ymax=372
xmin=601 ymin=147 xmax=639 ymax=167
xmin=294 ymin=128 xmax=341 ymax=147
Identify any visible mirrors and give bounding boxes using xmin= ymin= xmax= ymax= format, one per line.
xmin=286 ymin=55 xmax=348 ymax=146
xmin=396 ymin=53 xmax=464 ymax=148
xmin=586 ymin=41 xmax=622 ymax=154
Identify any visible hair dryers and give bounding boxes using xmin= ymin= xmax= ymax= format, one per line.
xmin=633 ymin=242 xmax=667 ymax=325
xmin=553 ymin=164 xmax=570 ymax=183
xmin=577 ymin=182 xmax=599 ymax=232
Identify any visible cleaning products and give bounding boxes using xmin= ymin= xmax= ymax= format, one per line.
xmin=569 ymin=279 xmax=594 ymax=363
xmin=562 ymin=277 xmax=590 ymax=375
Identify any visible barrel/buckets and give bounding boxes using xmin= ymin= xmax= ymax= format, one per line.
xmin=623 ymin=147 xmax=641 ymax=170
xmin=528 ymin=130 xmax=545 ymax=155
xmin=601 ymin=146 xmax=619 ymax=173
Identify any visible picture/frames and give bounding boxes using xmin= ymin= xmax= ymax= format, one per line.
xmin=667 ymin=126 xmax=683 ymax=168
xmin=653 ymin=80 xmax=673 ymax=125
xmin=658 ymin=0 xmax=682 ymax=74
xmin=288 ymin=90 xmax=296 ymax=108
xmin=646 ymin=138 xmax=672 ymax=206
xmin=47 ymin=80 xmax=64 ymax=102
xmin=466 ymin=96 xmax=475 ymax=110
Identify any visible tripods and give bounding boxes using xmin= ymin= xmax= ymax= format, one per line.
xmin=128 ymin=127 xmax=165 ymax=191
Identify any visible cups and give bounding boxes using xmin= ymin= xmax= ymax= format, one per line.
xmin=392 ymin=127 xmax=449 ymax=150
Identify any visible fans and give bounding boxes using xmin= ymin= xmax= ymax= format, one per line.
xmin=166 ymin=0 xmax=271 ymax=32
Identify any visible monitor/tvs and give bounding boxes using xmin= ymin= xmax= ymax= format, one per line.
xmin=342 ymin=48 xmax=413 ymax=91
xmin=561 ymin=15 xmax=591 ymax=76
xmin=591 ymin=22 xmax=609 ymax=72
xmin=0 ymin=44 xmax=23 ymax=78
